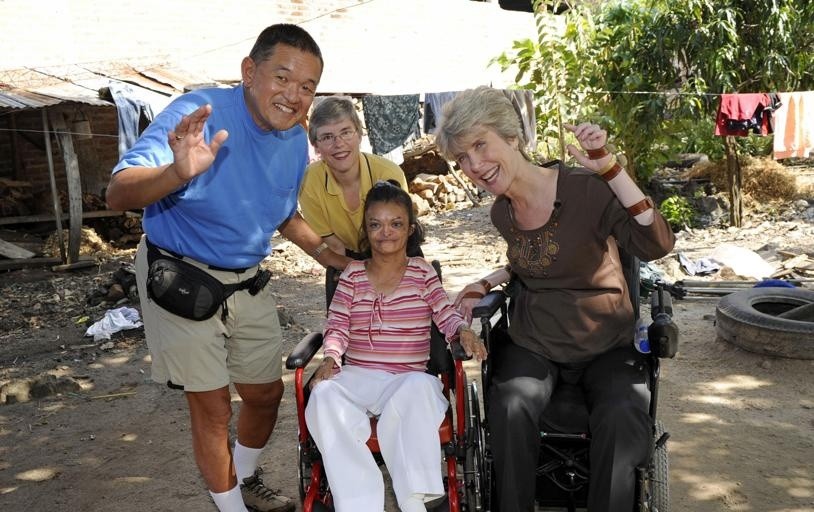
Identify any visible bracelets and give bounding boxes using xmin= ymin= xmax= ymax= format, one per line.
xmin=597 ymin=155 xmax=618 ymax=175
xmin=312 ymin=242 xmax=328 ymax=259
xmin=601 ymin=162 xmax=623 ymax=182
xmin=477 ymin=279 xmax=491 ymax=293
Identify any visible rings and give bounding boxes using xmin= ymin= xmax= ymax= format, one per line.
xmin=176 ymin=136 xmax=184 ymax=141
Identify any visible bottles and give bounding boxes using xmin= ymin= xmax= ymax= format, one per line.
xmin=634 ymin=304 xmax=656 ymax=353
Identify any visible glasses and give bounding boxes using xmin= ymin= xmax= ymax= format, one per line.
xmin=314 ymin=128 xmax=358 ymax=147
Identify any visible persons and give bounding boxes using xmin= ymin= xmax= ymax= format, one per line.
xmin=434 ymin=87 xmax=676 ymax=512
xmin=299 ymin=98 xmax=424 ymax=262
xmin=305 ymin=180 xmax=488 ymax=512
xmin=106 ymin=24 xmax=356 ymax=512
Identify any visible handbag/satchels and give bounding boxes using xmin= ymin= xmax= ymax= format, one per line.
xmin=146 ymin=255 xmax=229 ymax=322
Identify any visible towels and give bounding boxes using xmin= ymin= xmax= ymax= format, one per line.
xmin=773 ymin=90 xmax=814 ymax=160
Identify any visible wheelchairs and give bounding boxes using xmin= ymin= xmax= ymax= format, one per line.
xmin=467 ymin=270 xmax=687 ymax=510
xmin=283 ymin=256 xmax=486 ymax=511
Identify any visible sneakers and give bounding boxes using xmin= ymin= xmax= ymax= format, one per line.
xmin=238 ymin=466 xmax=296 ymax=512
